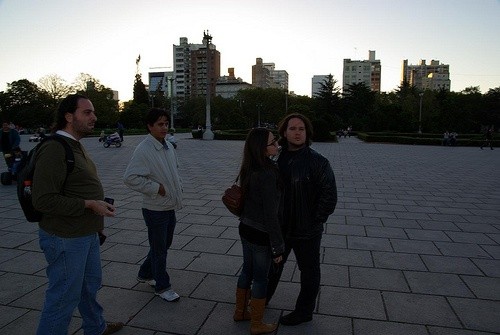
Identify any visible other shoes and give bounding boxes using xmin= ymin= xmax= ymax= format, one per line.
xmin=101 ymin=321 xmax=123 ymax=335
xmin=247 ymin=296 xmax=270 ymax=307
xmin=279 ymin=308 xmax=313 ymax=326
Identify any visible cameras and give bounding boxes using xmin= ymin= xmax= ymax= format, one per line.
xmin=104 ymin=197 xmax=114 ymax=211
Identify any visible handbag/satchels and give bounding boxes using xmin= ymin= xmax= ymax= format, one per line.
xmin=221 ymin=175 xmax=243 ymax=217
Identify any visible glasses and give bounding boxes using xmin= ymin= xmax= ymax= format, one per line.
xmin=266 ymin=140 xmax=278 ymax=146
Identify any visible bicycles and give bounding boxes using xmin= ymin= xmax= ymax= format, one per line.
xmin=103 ymin=134 xmax=122 ymax=148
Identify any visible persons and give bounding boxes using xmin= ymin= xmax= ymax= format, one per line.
xmin=11 ymin=147 xmax=23 ymax=176
xmin=198 ymin=124 xmax=203 ymax=139
xmin=0 ymin=120 xmax=20 ymax=172
xmin=111 ymin=130 xmax=120 ymax=145
xmin=100 ymin=130 xmax=105 ymax=138
xmin=265 ymin=114 xmax=337 ymax=327
xmin=442 ymin=130 xmax=457 ymax=146
xmin=232 ymin=128 xmax=285 ymax=335
xmin=480 ymin=124 xmax=495 ymax=150
xmin=124 ymin=109 xmax=184 ymax=302
xmin=338 ymin=126 xmax=352 ymax=138
xmin=31 ymin=94 xmax=124 ymax=335
xmin=39 ymin=125 xmax=45 ymax=143
xmin=170 ymin=127 xmax=175 ymax=136
xmin=117 ymin=122 xmax=124 ymax=142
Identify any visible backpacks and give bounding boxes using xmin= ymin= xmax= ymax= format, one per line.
xmin=17 ymin=134 xmax=76 ymax=222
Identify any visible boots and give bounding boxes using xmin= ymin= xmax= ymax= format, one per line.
xmin=234 ymin=288 xmax=252 ymax=321
xmin=249 ymin=303 xmax=277 ymax=335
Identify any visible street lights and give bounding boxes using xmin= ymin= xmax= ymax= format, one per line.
xmin=255 ymin=104 xmax=263 ymax=127
xmin=237 ymin=98 xmax=245 ymax=108
xmin=418 ymin=93 xmax=424 ymax=134
xmin=284 ymin=91 xmax=288 ymax=113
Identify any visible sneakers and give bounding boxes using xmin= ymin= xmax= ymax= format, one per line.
xmin=153 ymin=287 xmax=180 ymax=302
xmin=137 ymin=276 xmax=157 ymax=287
